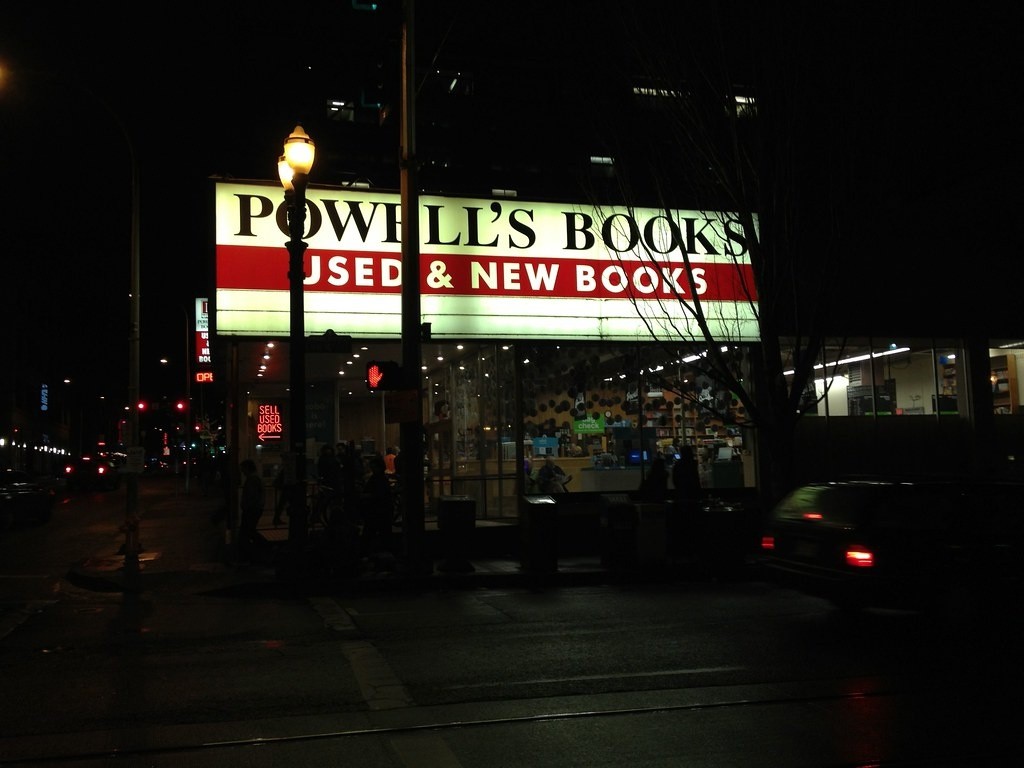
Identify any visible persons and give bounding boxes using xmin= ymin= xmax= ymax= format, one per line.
xmin=515 ymin=455 xmax=570 ymax=495
xmin=234 ymin=440 xmax=431 ymax=565
xmin=641 ymin=446 xmax=703 ymax=492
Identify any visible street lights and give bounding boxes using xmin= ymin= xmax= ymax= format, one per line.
xmin=274 ymin=126 xmax=320 ymax=604
xmin=0 ymin=64 xmax=145 ymax=635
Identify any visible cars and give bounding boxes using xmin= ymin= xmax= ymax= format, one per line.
xmin=0 ymin=470 xmax=47 ymax=530
xmin=756 ymin=478 xmax=1024 ymax=619
xmin=64 ymin=455 xmax=121 ymax=488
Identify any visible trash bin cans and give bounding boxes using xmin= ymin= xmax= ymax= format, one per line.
xmin=599 ymin=502 xmax=640 ymax=567
xmin=437 ymin=495 xmax=477 ymax=558
xmin=522 ymin=495 xmax=559 ymax=572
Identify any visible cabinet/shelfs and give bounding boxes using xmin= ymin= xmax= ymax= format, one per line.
xmin=640 ymin=390 xmax=743 ymax=447
